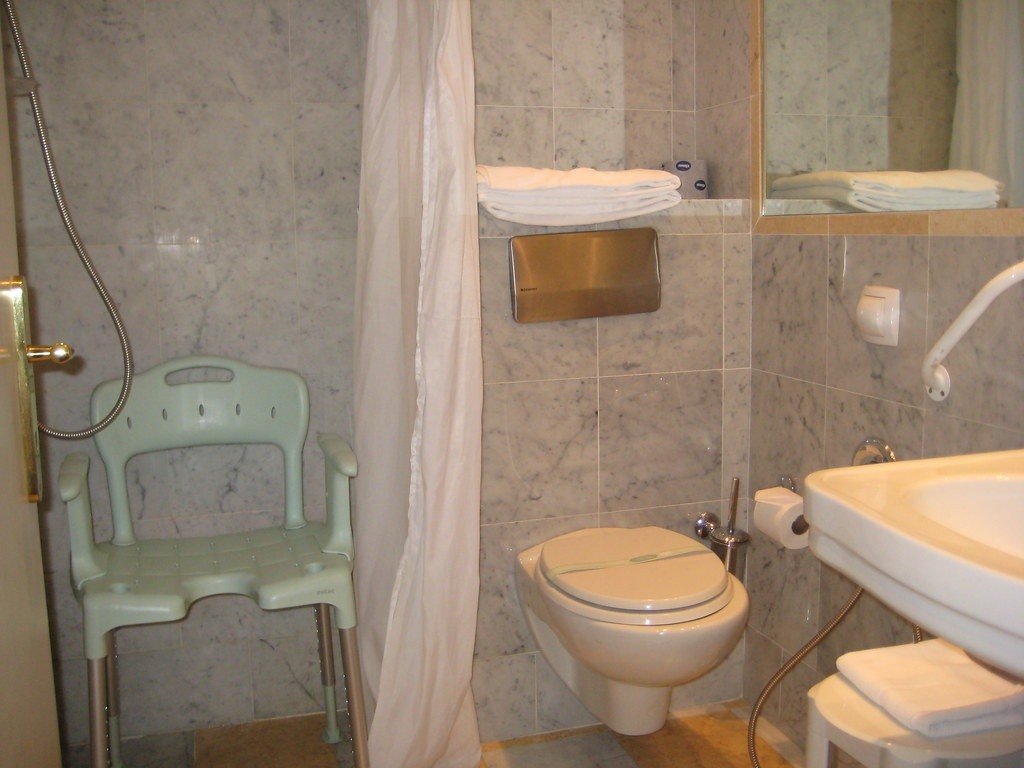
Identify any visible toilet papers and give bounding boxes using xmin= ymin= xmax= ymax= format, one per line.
xmin=753 ymin=486 xmax=809 ymax=549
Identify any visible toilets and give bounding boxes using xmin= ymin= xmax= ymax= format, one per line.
xmin=514 ymin=525 xmax=748 ymax=735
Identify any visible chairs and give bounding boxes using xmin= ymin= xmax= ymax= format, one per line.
xmin=59 ymin=354 xmax=371 ymax=768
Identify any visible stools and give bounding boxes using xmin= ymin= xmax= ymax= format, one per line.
xmin=806 ymin=671 xmax=1024 ymax=768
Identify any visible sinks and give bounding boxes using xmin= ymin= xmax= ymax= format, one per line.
xmin=804 ymin=444 xmax=1023 ymax=680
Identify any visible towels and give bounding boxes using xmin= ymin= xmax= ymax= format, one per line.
xmin=475 ymin=164 xmax=681 ymax=227
xmin=768 ymin=170 xmax=1003 ymax=212
xmin=835 ymin=637 xmax=1024 ymax=739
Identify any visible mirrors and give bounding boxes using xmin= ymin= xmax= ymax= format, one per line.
xmin=750 ymin=0 xmax=1024 ymax=238
